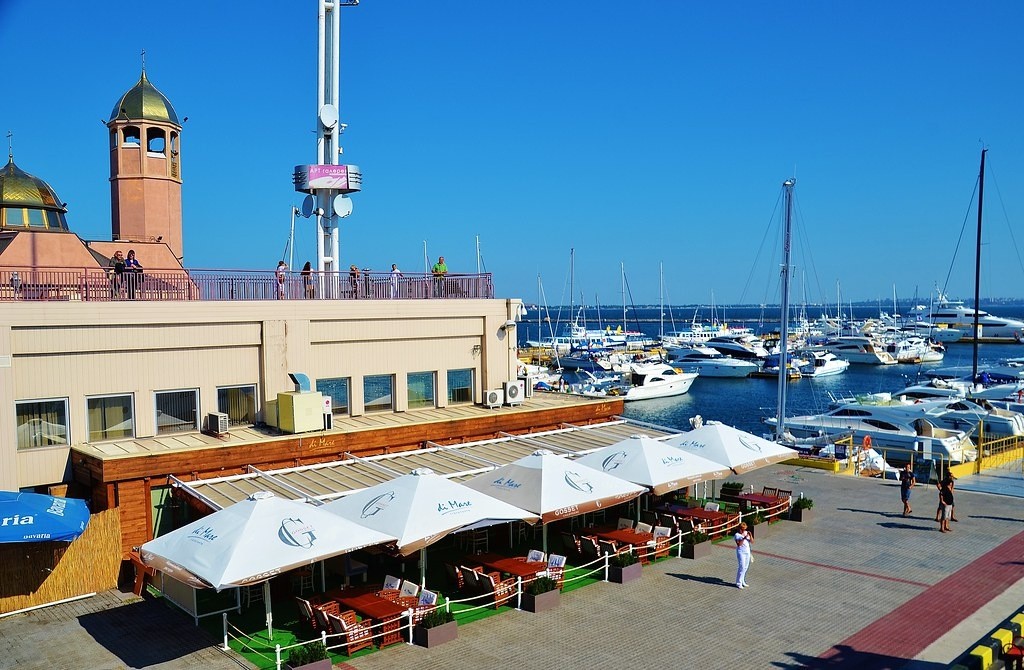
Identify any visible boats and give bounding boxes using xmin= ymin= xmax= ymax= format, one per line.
xmin=572 ymin=336 xmax=946 ymax=401
xmin=902 ymin=281 xmax=1024 ymax=341
xmin=921 ymin=356 xmax=1024 ymax=381
xmin=763 ymin=389 xmax=1024 ymax=464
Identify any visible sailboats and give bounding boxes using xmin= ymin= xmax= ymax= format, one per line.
xmin=690 ymin=175 xmax=917 ymax=486
xmin=517 ymin=246 xmax=963 ymax=349
xmin=903 ymin=137 xmax=1024 ymax=405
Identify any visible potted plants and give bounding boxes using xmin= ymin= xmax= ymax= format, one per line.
xmin=747 ymin=514 xmax=768 ymax=538
xmin=720 ymin=480 xmax=745 ymax=502
xmin=683 ymin=531 xmax=712 ymax=559
xmin=611 ymin=553 xmax=642 ymax=583
xmin=791 ymin=497 xmax=816 ymax=521
xmin=283 ymin=641 xmax=331 ymax=670
xmin=418 ymin=610 xmax=458 ymax=648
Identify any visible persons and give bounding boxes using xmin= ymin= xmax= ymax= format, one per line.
xmin=349 ymin=265 xmax=359 ymax=299
xmin=300 ymin=262 xmax=317 ymax=300
xmin=935 ymin=472 xmax=959 ymax=533
xmin=431 ymin=257 xmax=448 ymax=299
xmin=899 ymin=464 xmax=915 ymax=517
xmin=734 ymin=523 xmax=754 ymax=590
xmin=109 ymin=250 xmax=141 ymax=301
xmin=389 ymin=264 xmax=403 ymax=298
xmin=275 ymin=261 xmax=288 ymax=299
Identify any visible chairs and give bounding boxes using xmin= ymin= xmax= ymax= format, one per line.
xmin=238 ymin=485 xmax=792 ymax=657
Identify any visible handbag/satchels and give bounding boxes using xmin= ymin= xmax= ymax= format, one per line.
xmin=750 ymin=554 xmax=754 ymax=562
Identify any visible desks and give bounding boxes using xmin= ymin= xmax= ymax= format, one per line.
xmin=737 ymin=495 xmax=780 ymax=523
xmin=465 ymin=553 xmax=543 ymax=593
xmin=331 ymin=588 xmax=407 ymax=645
xmin=584 ymin=526 xmax=651 ymax=563
xmin=654 ymin=505 xmax=727 ymax=541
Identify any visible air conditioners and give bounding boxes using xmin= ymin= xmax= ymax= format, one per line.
xmin=208 ymin=412 xmax=228 ymax=434
xmin=484 ymin=376 xmax=533 ymax=406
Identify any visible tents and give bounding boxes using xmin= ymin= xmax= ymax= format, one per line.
xmin=0 ymin=490 xmax=90 ymax=544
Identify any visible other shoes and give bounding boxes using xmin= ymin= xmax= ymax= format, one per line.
xmin=903 ymin=510 xmax=912 ymax=518
xmin=944 ymin=527 xmax=951 ymax=531
xmin=736 ymin=585 xmax=743 ymax=589
xmin=951 ymin=518 xmax=958 ymax=522
xmin=940 ymin=529 xmax=946 ymax=533
xmin=743 ymin=583 xmax=749 ymax=587
xmin=935 ymin=518 xmax=940 ymax=522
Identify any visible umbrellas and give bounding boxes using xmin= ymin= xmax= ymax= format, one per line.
xmin=663 ymin=420 xmax=799 ymax=502
xmin=105 ymin=409 xmax=192 ymax=431
xmin=315 ymin=468 xmax=541 ymax=590
xmin=17 ymin=419 xmax=66 ymax=448
xmin=571 ymin=434 xmax=731 ymax=522
xmin=364 ymin=389 xmax=429 ymax=406
xmin=460 ymin=449 xmax=649 ymax=554
xmin=139 ymin=492 xmax=398 ymax=640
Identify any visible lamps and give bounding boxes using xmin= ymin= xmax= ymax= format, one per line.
xmin=101 ymin=120 xmax=108 ymax=124
xmin=121 ymin=108 xmax=130 ymax=122
xmin=501 ymin=321 xmax=516 ymax=331
xmin=525 ymin=577 xmax=560 ymax=613
xmin=62 ymin=203 xmax=67 ymax=207
xmin=179 ymin=117 xmax=188 ymax=127
xmin=158 ymin=236 xmax=162 ymax=241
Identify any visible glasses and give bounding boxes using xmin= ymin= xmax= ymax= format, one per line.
xmin=116 ymin=254 xmax=122 ymax=256
